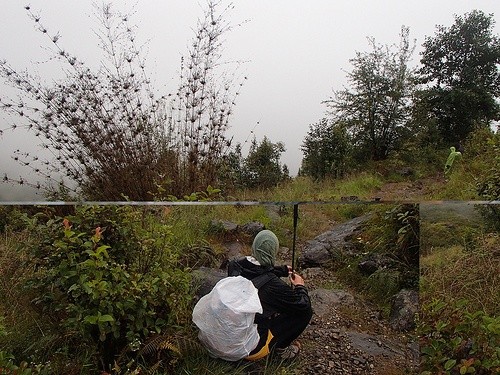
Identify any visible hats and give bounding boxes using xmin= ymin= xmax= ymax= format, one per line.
xmin=251 ymin=230 xmax=279 ymax=269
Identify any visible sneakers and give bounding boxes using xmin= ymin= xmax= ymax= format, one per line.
xmin=272 ymin=344 xmax=300 ymax=360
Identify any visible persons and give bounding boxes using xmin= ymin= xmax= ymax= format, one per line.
xmin=228 ymin=229 xmax=314 ymax=363
xmin=445 ymin=146 xmax=462 ymax=168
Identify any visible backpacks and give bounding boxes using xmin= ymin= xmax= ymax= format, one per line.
xmin=192 ymin=262 xmax=276 ymax=359
